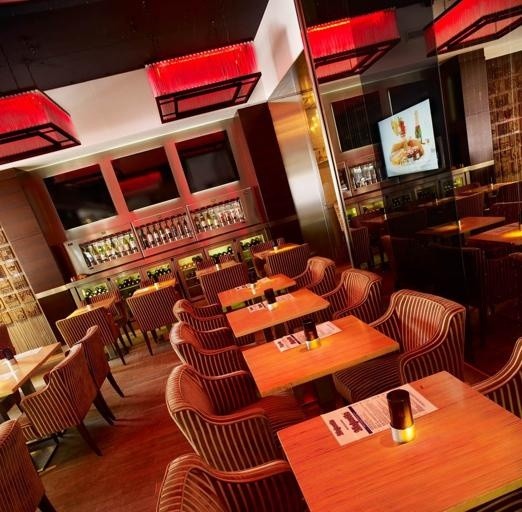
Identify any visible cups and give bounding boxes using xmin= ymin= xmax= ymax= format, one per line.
xmin=391 ymin=117 xmax=407 ymax=139
xmin=3 ymin=347 xmax=18 ymax=367
xmin=386 ymin=387 xmax=418 ymax=443
xmin=248 ymin=267 xmax=321 ymax=353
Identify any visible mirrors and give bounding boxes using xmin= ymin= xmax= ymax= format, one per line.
xmin=292 ymin=1 xmax=522 ymax=378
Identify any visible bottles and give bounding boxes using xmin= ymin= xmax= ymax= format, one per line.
xmin=79 ymin=199 xmax=244 ymax=268
xmin=347 ymin=183 xmax=456 ymax=222
xmin=83 ymin=236 xmax=263 ymax=299
xmin=413 ymin=110 xmax=423 ymax=143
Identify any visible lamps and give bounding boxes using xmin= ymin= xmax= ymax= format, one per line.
xmin=0 ymin=35 xmax=82 ymax=163
xmin=142 ymin=21 xmax=263 ymax=123
xmin=307 ymin=9 xmax=404 ymax=85
xmin=420 ymin=1 xmax=519 ymax=58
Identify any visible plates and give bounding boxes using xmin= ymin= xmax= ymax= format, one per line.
xmin=388 ymin=142 xmax=430 ymax=174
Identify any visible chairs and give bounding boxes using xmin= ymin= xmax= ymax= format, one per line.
xmin=344 ymin=178 xmax=520 ymax=225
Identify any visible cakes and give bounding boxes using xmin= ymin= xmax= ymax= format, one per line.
xmin=391 ymin=140 xmax=424 ymax=164
